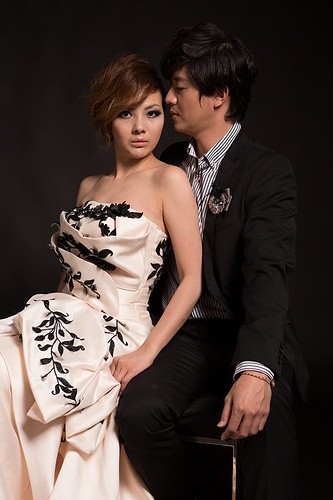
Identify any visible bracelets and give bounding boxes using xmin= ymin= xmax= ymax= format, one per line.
xmin=241 ymin=370 xmax=275 ymax=385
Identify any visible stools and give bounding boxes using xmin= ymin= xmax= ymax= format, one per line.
xmin=181 ymin=429 xmax=243 ymax=500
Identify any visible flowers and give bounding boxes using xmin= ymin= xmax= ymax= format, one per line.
xmin=206 ymin=182 xmax=232 ymax=214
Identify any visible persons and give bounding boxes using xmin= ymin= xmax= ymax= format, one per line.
xmin=0 ymin=56 xmax=203 ymax=500
xmin=114 ymin=23 xmax=299 ymax=499
